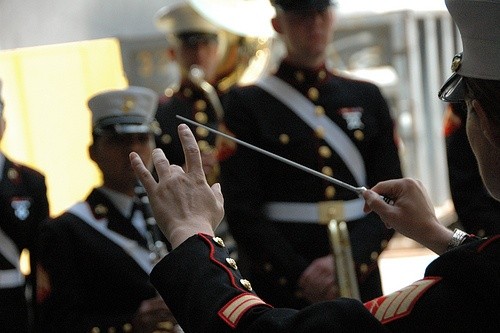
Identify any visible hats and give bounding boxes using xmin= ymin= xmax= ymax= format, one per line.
xmin=155 ymin=1 xmax=220 ymax=35
xmin=87 ymin=88 xmax=162 ymax=136
xmin=437 ymin=0 xmax=500 ymax=103
xmin=268 ymin=0 xmax=341 ymax=14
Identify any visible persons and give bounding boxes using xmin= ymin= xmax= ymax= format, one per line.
xmin=445 ymin=59 xmax=500 ymax=237
xmin=0 ymin=0 xmax=403 ymax=333
xmin=129 ymin=0 xmax=500 ymax=333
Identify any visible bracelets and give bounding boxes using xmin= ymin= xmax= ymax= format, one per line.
xmin=445 ymin=228 xmax=471 ymax=253
